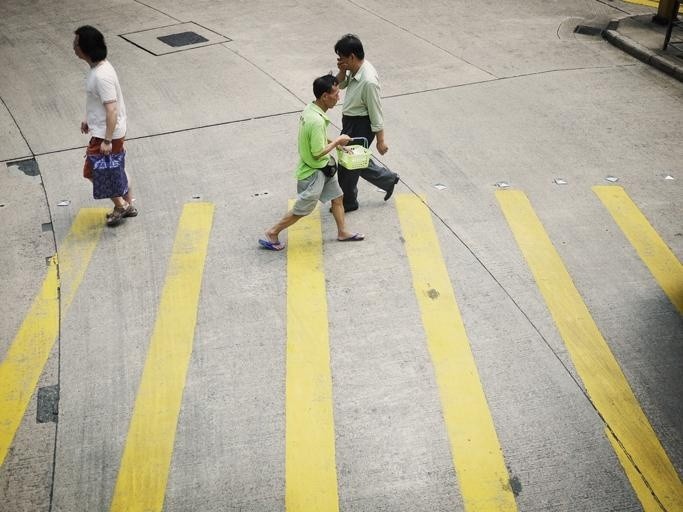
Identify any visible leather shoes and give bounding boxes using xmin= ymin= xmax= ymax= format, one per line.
xmin=383 ymin=176 xmax=399 ymax=202
xmin=327 ymin=204 xmax=358 ymax=214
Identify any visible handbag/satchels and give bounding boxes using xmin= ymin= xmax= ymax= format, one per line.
xmin=85 ymin=147 xmax=128 ymax=201
xmin=320 ymin=153 xmax=338 ymax=180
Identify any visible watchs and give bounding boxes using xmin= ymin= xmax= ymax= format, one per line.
xmin=103 ymin=137 xmax=112 ymax=143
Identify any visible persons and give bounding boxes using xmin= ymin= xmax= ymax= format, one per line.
xmin=255 ymin=74 xmax=364 ymax=252
xmin=329 ymin=34 xmax=400 ymax=215
xmin=70 ymin=24 xmax=138 ymax=228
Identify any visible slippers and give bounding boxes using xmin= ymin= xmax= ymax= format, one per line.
xmin=256 ymin=238 xmax=287 ymax=251
xmin=336 ymin=229 xmax=365 ymax=243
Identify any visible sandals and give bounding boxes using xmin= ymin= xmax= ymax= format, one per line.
xmin=103 ymin=197 xmax=137 ymax=226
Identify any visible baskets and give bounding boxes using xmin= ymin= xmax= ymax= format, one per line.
xmin=334 ymin=134 xmax=371 ymax=171
